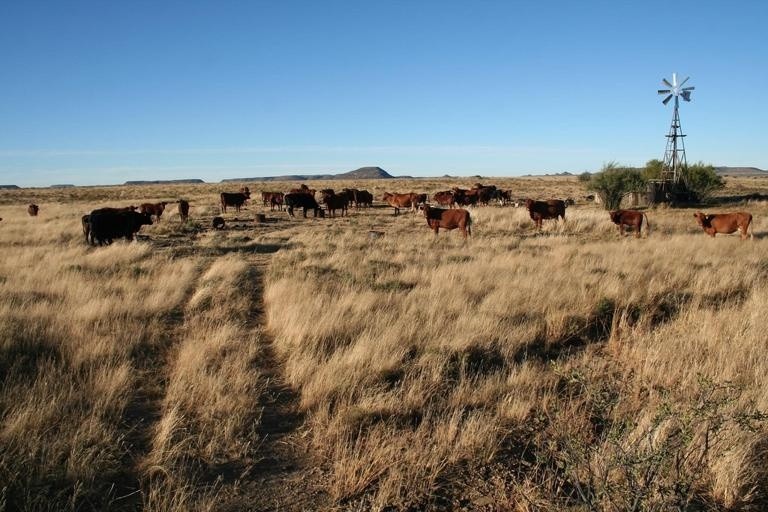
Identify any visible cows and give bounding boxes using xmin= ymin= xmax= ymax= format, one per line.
xmin=433 ymin=183 xmax=512 ymax=209
xmin=27 ymin=204 xmax=39 ymax=216
xmin=419 ymin=203 xmax=472 ymax=238
xmin=693 ymin=210 xmax=756 ymax=240
xmin=382 ymin=191 xmax=418 ymax=216
xmin=418 ymin=193 xmax=430 ymax=205
xmin=138 ymin=200 xmax=169 ymax=220
xmin=219 ymin=191 xmax=251 ymax=214
xmin=176 ymin=199 xmax=189 ymax=222
xmin=518 ymin=198 xmax=570 ymax=232
xmin=260 ymin=188 xmax=374 ymax=219
xmin=81 ymin=201 xmax=154 ymax=246
xmin=608 ymin=210 xmax=650 ymax=237
xmin=238 ymin=187 xmax=249 ymax=207
